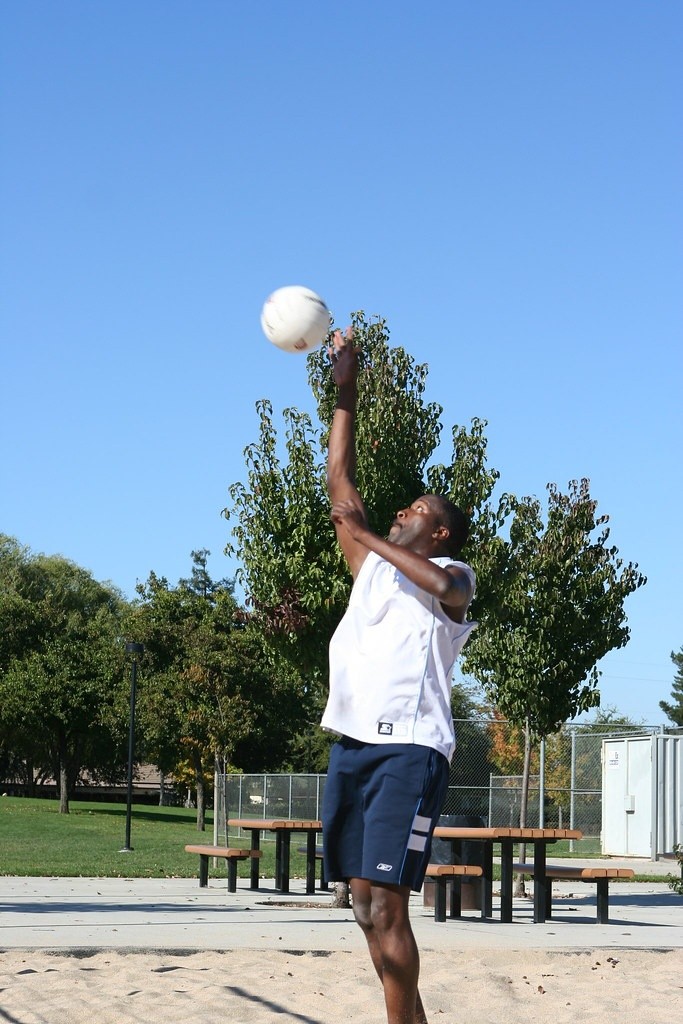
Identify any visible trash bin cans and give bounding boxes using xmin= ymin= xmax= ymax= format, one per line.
xmin=424 ymin=813 xmax=494 ymax=911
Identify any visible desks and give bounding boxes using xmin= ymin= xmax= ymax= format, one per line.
xmin=227 ymin=819 xmax=322 ymax=894
xmin=432 ymin=827 xmax=582 ymax=924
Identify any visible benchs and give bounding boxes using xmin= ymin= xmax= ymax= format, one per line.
xmin=297 ymin=847 xmax=328 ymax=891
xmin=186 ymin=844 xmax=262 ymax=893
xmin=425 ymin=863 xmax=482 ymax=922
xmin=514 ymin=862 xmax=634 ymax=924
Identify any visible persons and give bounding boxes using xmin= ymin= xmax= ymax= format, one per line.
xmin=318 ymin=327 xmax=478 ymax=1024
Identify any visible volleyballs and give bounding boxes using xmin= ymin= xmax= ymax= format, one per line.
xmin=259 ymin=285 xmax=333 ymax=357
